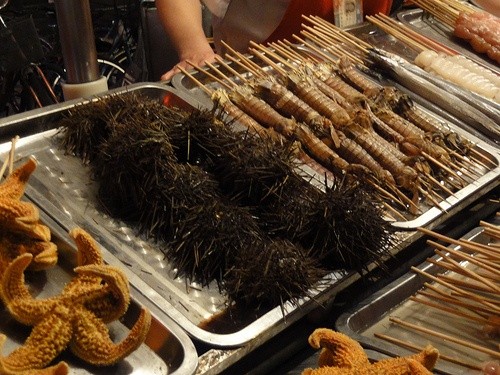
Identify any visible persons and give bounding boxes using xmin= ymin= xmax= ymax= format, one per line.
xmin=153 ymin=0 xmax=399 ymax=80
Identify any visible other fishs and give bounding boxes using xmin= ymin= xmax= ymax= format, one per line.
xmin=359 ymin=47 xmax=500 ymax=151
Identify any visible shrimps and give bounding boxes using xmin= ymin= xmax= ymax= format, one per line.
xmin=210 ymin=53 xmax=473 ymax=200
